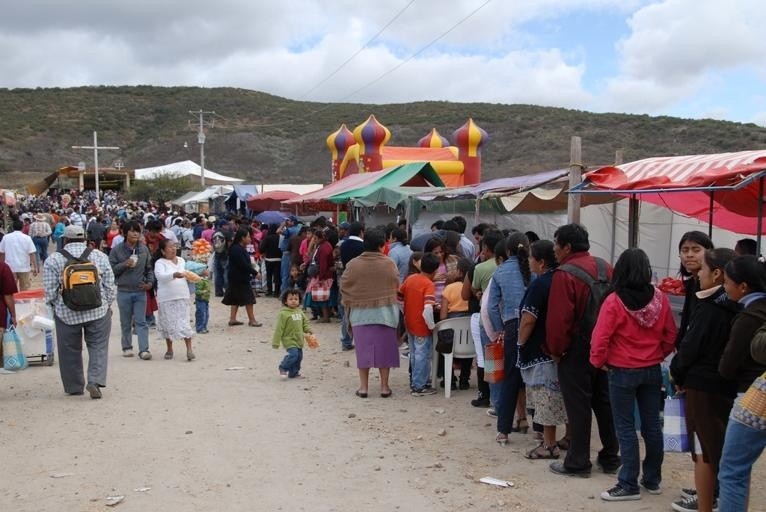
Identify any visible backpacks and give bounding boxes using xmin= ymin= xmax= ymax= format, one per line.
xmin=56 ymin=247 xmax=102 ymax=312
xmin=553 ymin=258 xmax=614 ymax=342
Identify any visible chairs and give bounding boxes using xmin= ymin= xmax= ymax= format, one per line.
xmin=429 ymin=316 xmax=480 ymax=396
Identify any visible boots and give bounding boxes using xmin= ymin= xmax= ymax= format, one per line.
xmin=471 ymin=367 xmax=490 ymax=407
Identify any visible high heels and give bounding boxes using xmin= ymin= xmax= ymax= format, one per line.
xmin=486 ymin=406 xmax=544 ymax=447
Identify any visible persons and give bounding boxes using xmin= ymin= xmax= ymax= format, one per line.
xmin=515 ymin=240 xmax=571 ymax=459
xmin=590 ymin=247 xmax=676 ymax=502
xmin=273 ymin=216 xmax=539 ymax=445
xmin=0 ymin=189 xmax=281 ymax=398
xmin=669 ymin=231 xmax=766 ymax=510
xmin=550 ymin=224 xmax=622 ymax=478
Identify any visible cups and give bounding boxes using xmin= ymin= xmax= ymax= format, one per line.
xmin=130 ymin=257 xmax=138 ymax=268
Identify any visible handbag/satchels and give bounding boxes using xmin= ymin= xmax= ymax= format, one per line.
xmin=281 ymin=238 xmax=290 ymax=251
xmin=312 ymin=286 xmax=329 ymax=301
xmin=2 ymin=325 xmax=29 ymax=372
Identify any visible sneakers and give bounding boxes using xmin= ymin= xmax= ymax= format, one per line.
xmin=248 ymin=322 xmax=262 ymax=326
xmin=187 ymin=353 xmax=196 ymax=361
xmin=164 ymin=352 xmax=173 ymax=359
xmin=401 ymin=342 xmax=410 ymax=360
xmin=380 ymin=390 xmax=391 ymax=397
xmin=279 ymin=365 xmax=287 ymax=375
xmin=140 ymin=351 xmax=152 ymax=360
xmin=601 ymin=478 xmax=662 ymax=501
xmin=670 ymin=488 xmax=719 ymax=512
xmin=292 ymin=372 xmax=304 ymax=379
xmin=356 ymin=389 xmax=367 ymax=397
xmin=228 ymin=321 xmax=243 ymax=326
xmin=410 ymin=376 xmax=470 ymax=395
xmin=123 ymin=350 xmax=134 ymax=357
xmin=342 ymin=342 xmax=356 ymax=351
xmin=310 ymin=313 xmax=340 ymax=323
xmin=198 ymin=329 xmax=208 ymax=334
xmin=87 ymin=382 xmax=102 ymax=398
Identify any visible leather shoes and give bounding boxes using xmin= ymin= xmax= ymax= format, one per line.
xmin=549 ymin=455 xmax=617 ymax=477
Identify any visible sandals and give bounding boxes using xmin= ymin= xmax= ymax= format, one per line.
xmin=522 ymin=437 xmax=570 ymax=459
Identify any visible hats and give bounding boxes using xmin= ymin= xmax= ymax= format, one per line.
xmin=285 ymin=216 xmax=298 ymax=223
xmin=219 ymin=219 xmax=229 ymax=224
xmin=59 ymin=225 xmax=85 ymax=239
xmin=33 ymin=213 xmax=46 ymax=221
xmin=337 ymin=222 xmax=350 ymax=228
